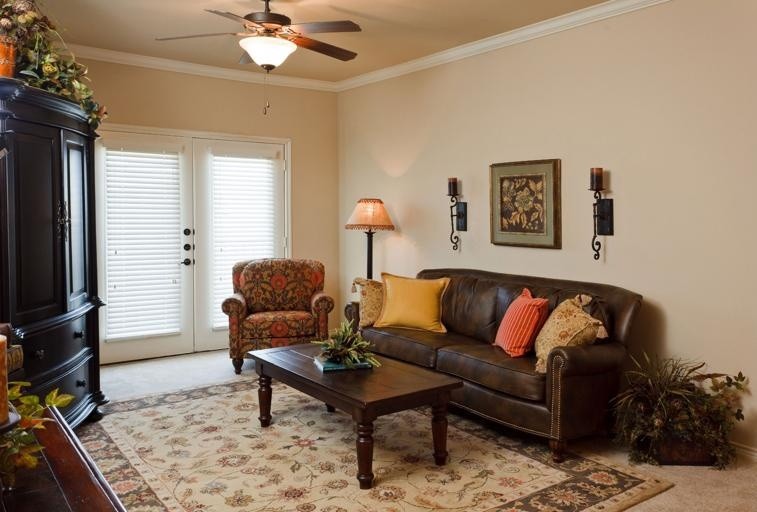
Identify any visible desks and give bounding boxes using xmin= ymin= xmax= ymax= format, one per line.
xmin=244 ymin=344 xmax=464 ymax=489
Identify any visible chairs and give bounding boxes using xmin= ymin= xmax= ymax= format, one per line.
xmin=220 ymin=256 xmax=334 ymax=373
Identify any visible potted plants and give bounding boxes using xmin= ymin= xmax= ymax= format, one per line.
xmin=610 ymin=347 xmax=752 ymax=475
xmin=0 ymin=0 xmax=104 ymax=113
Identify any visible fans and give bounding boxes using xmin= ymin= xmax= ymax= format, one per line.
xmin=147 ymin=1 xmax=362 ymax=70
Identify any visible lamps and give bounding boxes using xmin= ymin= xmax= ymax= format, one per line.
xmin=237 ymin=32 xmax=298 ymax=70
xmin=444 ymin=176 xmax=467 ymax=249
xmin=343 ymin=195 xmax=396 ymax=279
xmin=588 ymin=163 xmax=615 ymax=259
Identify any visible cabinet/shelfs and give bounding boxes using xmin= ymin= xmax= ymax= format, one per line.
xmin=0 ymin=398 xmax=123 ymax=512
xmin=0 ymin=77 xmax=105 ymax=436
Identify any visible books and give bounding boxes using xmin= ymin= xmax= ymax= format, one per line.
xmin=314 ymin=356 xmax=372 ymax=372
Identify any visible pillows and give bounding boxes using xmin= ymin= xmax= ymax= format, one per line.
xmin=488 ymin=283 xmax=549 ymax=358
xmin=372 ymin=271 xmax=450 ymax=336
xmin=533 ymin=291 xmax=612 ymax=371
xmin=350 ymin=277 xmax=383 ymax=329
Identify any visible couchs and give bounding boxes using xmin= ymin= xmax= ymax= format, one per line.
xmin=345 ymin=265 xmax=646 ymax=463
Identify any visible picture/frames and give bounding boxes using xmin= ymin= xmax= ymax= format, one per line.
xmin=487 ymin=156 xmax=561 ymax=252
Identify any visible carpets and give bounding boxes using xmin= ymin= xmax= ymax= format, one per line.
xmin=72 ymin=375 xmax=674 ymax=511
